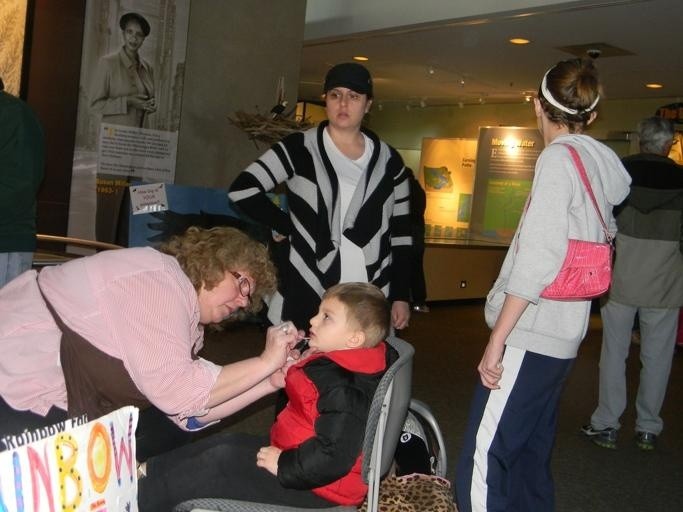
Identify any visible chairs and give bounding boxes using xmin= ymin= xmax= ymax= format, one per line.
xmin=172 ymin=335 xmax=447 ymax=512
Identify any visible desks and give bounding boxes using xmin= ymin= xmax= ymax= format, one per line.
xmin=421 ymin=238 xmax=511 ymax=304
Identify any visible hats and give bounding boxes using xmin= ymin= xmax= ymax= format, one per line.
xmin=320 ymin=61 xmax=372 ymax=96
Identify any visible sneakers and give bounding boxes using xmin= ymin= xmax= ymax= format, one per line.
xmin=575 ymin=415 xmax=620 ymax=449
xmin=634 ymin=427 xmax=658 ymax=452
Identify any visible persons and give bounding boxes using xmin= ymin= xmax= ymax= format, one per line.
xmin=453 ymin=52 xmax=633 ymax=512
xmin=0 ymin=221 xmax=316 ymax=446
xmin=87 ymin=12 xmax=158 ymax=252
xmin=224 ymin=60 xmax=429 ymax=421
xmin=138 ymin=281 xmax=397 ymax=511
xmin=578 ymin=116 xmax=683 ymax=451
xmin=0 ymin=77 xmax=50 ymax=289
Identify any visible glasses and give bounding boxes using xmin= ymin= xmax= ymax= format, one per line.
xmin=668 ymin=138 xmax=679 ymax=146
xmin=228 ymin=268 xmax=253 ymax=306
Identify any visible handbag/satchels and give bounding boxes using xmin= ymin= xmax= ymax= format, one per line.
xmin=513 ymin=233 xmax=613 ymax=302
